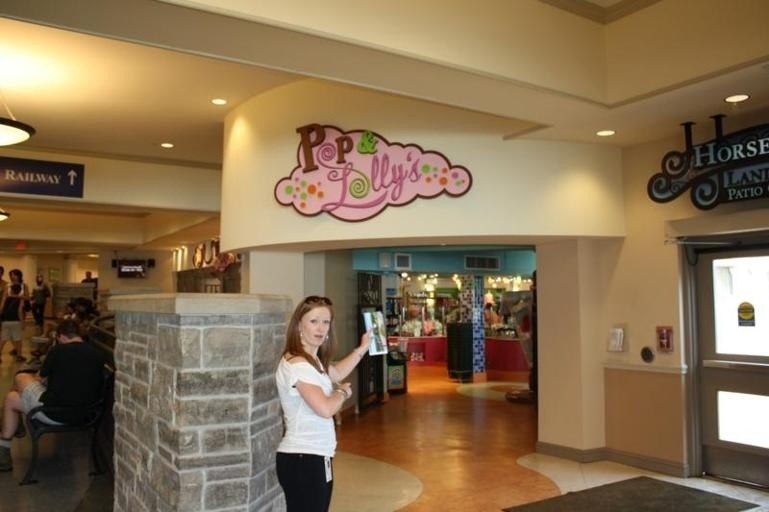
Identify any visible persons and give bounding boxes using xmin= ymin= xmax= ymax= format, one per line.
xmin=274 ymin=296 xmax=377 ymax=512
xmin=0 ymin=264 xmax=114 ymax=473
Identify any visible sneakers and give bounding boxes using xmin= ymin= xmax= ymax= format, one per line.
xmin=0 ymin=447 xmax=13 ymax=472
xmin=15 ymin=356 xmax=26 ymax=362
xmin=13 ymin=414 xmax=25 ymax=438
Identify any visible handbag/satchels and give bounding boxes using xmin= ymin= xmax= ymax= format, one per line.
xmin=23 ymin=300 xmax=31 ymax=313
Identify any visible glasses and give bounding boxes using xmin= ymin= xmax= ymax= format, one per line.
xmin=305 ymin=296 xmax=332 ymax=306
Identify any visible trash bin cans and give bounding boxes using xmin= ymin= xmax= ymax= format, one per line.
xmin=387 ymin=351 xmax=407 ymax=395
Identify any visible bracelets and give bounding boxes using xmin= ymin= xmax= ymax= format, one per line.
xmin=333 ymin=387 xmax=349 ymax=398
xmin=354 ymin=348 xmax=365 ymax=361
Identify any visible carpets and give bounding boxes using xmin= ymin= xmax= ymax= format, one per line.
xmin=500 ymin=475 xmax=761 ymax=512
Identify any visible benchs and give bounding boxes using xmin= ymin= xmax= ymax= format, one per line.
xmin=10 ymin=315 xmax=115 ymax=486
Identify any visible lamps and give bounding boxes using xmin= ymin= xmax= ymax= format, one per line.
xmin=0 ymin=97 xmax=36 ymax=147
